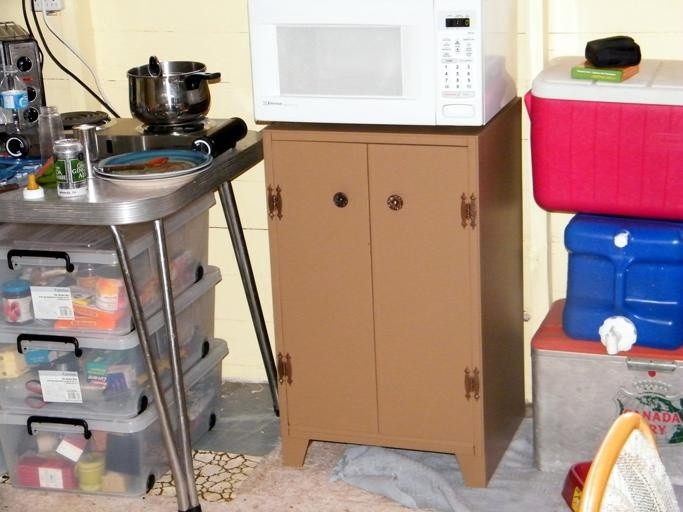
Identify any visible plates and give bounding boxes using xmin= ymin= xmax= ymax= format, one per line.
xmin=92 ymin=149 xmax=212 ymax=179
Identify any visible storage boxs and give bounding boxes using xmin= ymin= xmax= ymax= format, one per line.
xmin=2 ymin=191 xmax=218 ymax=334
xmin=524 ymin=55 xmax=683 ymax=487
xmin=1 ymin=337 xmax=228 ymax=497
xmin=1 ymin=264 xmax=224 ymax=418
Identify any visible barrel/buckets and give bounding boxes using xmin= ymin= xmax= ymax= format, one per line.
xmin=562 ymin=213 xmax=683 ymax=354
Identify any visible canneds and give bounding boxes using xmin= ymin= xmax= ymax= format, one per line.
xmin=53 ymin=138 xmax=89 ymax=198
xmin=0 ymin=278 xmax=33 ymax=326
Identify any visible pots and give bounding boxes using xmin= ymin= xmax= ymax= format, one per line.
xmin=126 ymin=55 xmax=221 ymax=127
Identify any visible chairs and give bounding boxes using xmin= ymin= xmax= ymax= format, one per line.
xmin=578 ymin=412 xmax=681 ymax=511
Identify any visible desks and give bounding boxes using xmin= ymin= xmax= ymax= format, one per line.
xmin=1 ymin=128 xmax=265 ymax=512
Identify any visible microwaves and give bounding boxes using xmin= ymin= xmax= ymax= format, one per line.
xmin=247 ymin=0 xmax=518 ymax=130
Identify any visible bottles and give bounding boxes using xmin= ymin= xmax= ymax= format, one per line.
xmin=39 ymin=108 xmax=66 ymax=163
xmin=0 ymin=63 xmax=30 ymax=134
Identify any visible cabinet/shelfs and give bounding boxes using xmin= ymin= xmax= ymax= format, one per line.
xmin=258 ymin=96 xmax=526 ymax=489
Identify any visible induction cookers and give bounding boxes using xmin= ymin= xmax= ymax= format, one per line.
xmin=87 ymin=116 xmax=248 ymax=154
